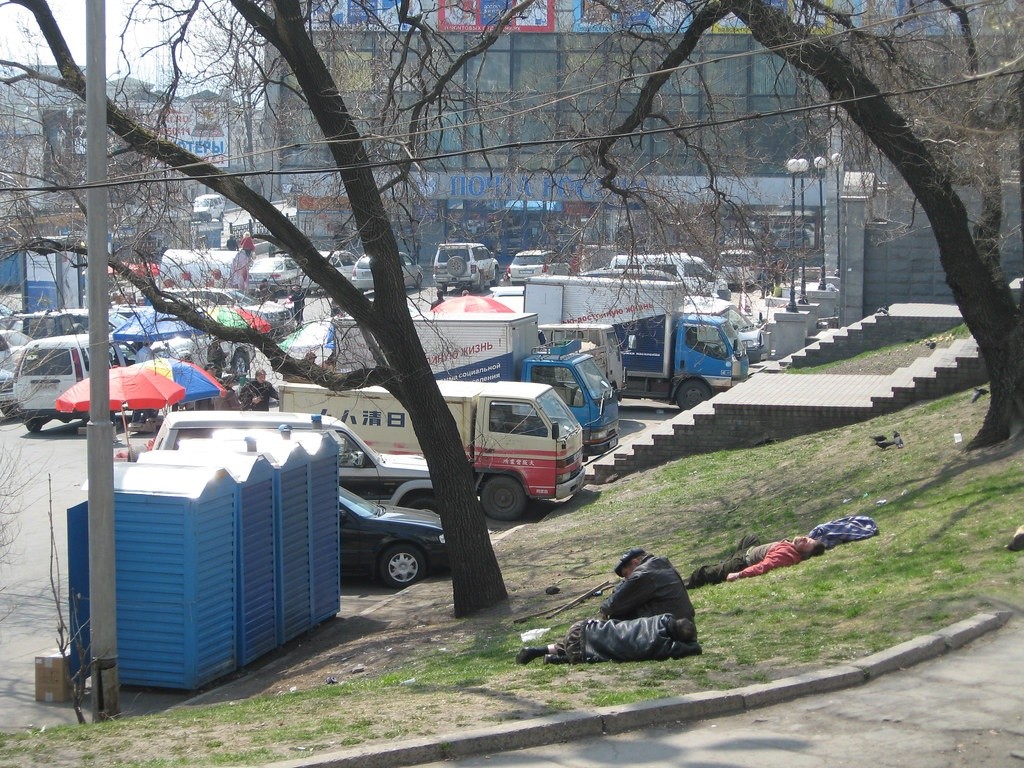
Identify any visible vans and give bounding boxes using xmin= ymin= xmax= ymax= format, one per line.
xmin=538 ymin=325 xmax=626 ymax=406
xmin=191 ymin=194 xmax=225 ymax=222
xmin=680 ymin=296 xmax=767 ymax=365
xmin=609 ymin=252 xmax=729 ymax=299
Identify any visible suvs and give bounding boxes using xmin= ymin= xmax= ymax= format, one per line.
xmin=128 ymin=412 xmax=440 ymax=513
xmin=432 ymin=243 xmax=499 ymax=293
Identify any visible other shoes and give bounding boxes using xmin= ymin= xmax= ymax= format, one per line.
xmin=515 ymin=646 xmax=532 ymax=665
xmin=544 ymin=654 xmax=554 ymax=665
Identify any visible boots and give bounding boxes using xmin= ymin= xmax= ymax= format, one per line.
xmin=113 ymin=425 xmax=123 ymax=443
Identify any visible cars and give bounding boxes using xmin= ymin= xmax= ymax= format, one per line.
xmin=504 ymin=249 xmax=572 ymax=286
xmin=339 ymin=486 xmax=447 ymax=588
xmin=249 ymin=259 xmax=301 ymax=293
xmin=350 ymin=251 xmax=424 ymax=294
xmin=0 ymin=288 xmax=291 ymax=432
xmin=580 ymin=272 xmax=711 ymax=299
xmin=713 ymin=249 xmax=764 ymax=290
xmin=299 ymin=251 xmax=361 ymax=293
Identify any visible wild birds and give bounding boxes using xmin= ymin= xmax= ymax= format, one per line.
xmin=926 ymin=339 xmax=936 ymax=349
xmin=971 ymin=385 xmax=990 ymax=402
xmin=867 ymin=429 xmax=904 ymax=450
xmin=877 ymin=303 xmax=889 ymax=315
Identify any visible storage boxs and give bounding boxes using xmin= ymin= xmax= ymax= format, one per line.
xmin=35 ymin=648 xmax=74 ymax=702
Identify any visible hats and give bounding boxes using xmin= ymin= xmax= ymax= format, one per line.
xmin=614 ymin=549 xmax=645 ymax=577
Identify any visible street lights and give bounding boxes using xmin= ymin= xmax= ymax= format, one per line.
xmin=785 ymin=156 xmax=803 ymax=318
xmin=833 ymin=152 xmax=846 ymax=282
xmin=811 ymin=157 xmax=831 ymax=293
xmin=796 ymin=159 xmax=810 ymax=307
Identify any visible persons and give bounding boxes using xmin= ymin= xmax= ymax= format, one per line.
xmin=65 ymin=323 xmax=85 ymax=377
xmin=431 ymin=289 xmax=445 ymax=310
xmin=760 ymin=260 xmax=787 ymax=299
xmin=515 ymin=612 xmax=703 ymax=666
xmin=681 ymin=533 xmax=825 ymax=589
xmin=226 ymin=234 xmax=239 ymax=251
xmin=462 ymin=290 xmax=469 ymax=297
xmin=241 ymin=232 xmax=255 ymax=256
xmin=239 ymin=368 xmax=279 ymax=411
xmin=214 ymin=375 xmax=254 ymax=410
xmin=597 ymin=548 xmax=695 ymax=621
xmin=260 ymin=278 xmax=278 ymax=302
xmin=285 ymin=284 xmax=306 ymax=324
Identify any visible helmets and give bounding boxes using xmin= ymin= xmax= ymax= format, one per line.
xmin=305 ymin=351 xmax=317 ymax=360
xmin=255 ymin=369 xmax=267 ymax=375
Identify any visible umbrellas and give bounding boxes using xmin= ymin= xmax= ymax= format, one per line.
xmin=432 ymin=294 xmax=516 ymax=313
xmin=112 ymin=305 xmax=271 ymax=342
xmin=56 ymin=357 xmax=227 ymax=462
xmin=82 ymin=259 xmax=160 ymax=278
xmin=276 ymin=322 xmax=335 ymax=365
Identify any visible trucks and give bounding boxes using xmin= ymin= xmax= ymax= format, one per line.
xmin=279 ymin=381 xmax=587 ymax=521
xmin=337 ymin=313 xmax=619 ymax=452
xmin=522 ymin=274 xmax=751 ymax=411
